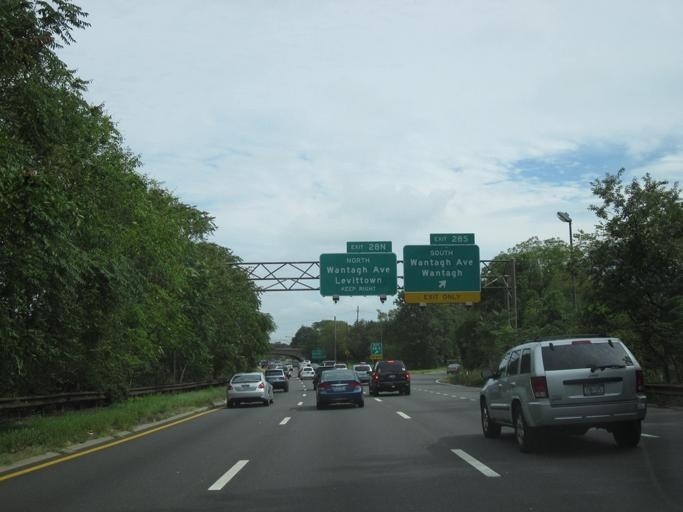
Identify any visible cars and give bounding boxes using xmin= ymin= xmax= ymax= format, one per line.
xmin=259 ymin=358 xmax=346 ymax=379
xmin=227 ymin=372 xmax=274 ymax=408
xmin=367 ymin=360 xmax=410 ymax=395
xmin=264 ymin=368 xmax=288 ymax=392
xmin=316 ymin=369 xmax=363 ymax=408
xmin=312 ymin=367 xmax=336 ymax=390
xmin=446 ymin=363 xmax=461 ymax=374
xmin=352 ymin=362 xmax=371 ymax=385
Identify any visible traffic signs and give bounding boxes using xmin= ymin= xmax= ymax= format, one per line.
xmin=403 ymin=245 xmax=481 ymax=305
xmin=320 ymin=252 xmax=397 ymax=296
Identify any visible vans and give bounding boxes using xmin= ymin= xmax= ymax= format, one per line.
xmin=478 ymin=335 xmax=647 ymax=453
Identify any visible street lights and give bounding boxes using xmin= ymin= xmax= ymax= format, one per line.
xmin=556 ymin=210 xmax=576 ymax=311
